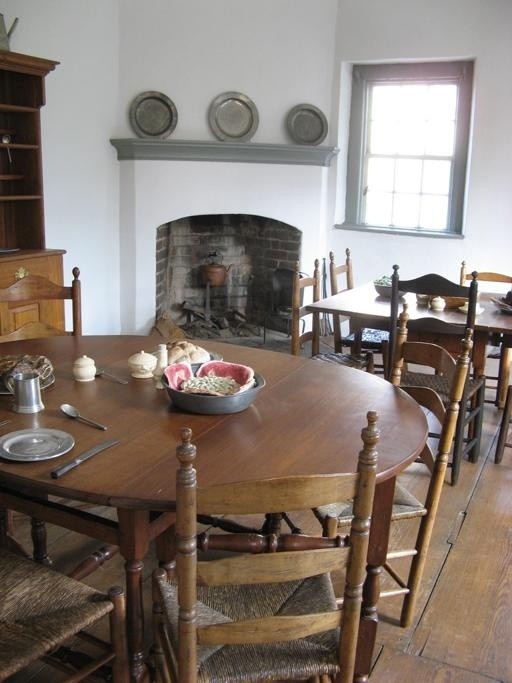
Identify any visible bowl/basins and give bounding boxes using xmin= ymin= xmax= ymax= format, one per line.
xmin=373 ymin=278 xmax=409 ymax=297
xmin=161 ymin=362 xmax=267 ymax=414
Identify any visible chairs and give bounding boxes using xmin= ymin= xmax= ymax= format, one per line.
xmin=458 ymin=259 xmax=512 ymax=306
xmin=329 ymin=247 xmax=391 ymax=376
xmin=1 ymin=265 xmax=83 ymax=343
xmin=153 ymin=409 xmax=381 ymax=683
xmin=290 ymin=257 xmax=375 ymax=376
xmin=283 ymin=311 xmax=472 ymax=628
xmin=387 ymin=262 xmax=487 ymax=487
xmin=0 ymin=544 xmax=133 ymax=683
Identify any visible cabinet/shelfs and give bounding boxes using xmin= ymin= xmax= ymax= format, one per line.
xmin=1 ymin=49 xmax=67 ymax=339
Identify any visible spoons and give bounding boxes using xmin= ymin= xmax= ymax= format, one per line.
xmin=59 ymin=403 xmax=110 ymax=431
xmin=95 ymin=367 xmax=131 ymax=385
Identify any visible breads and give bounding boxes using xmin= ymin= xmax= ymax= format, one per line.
xmin=165 ymin=340 xmax=211 ymax=365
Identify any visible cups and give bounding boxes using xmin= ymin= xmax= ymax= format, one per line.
xmin=4 ymin=372 xmax=46 ymax=414
xmin=465 ymin=301 xmax=480 ymax=310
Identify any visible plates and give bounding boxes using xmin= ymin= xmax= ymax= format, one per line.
xmin=0 ymin=427 xmax=75 ymax=461
xmin=284 ymin=102 xmax=330 ymax=145
xmin=147 ymin=349 xmax=224 ymax=362
xmin=458 ymin=305 xmax=484 ymax=314
xmin=129 ymin=88 xmax=179 ymax=140
xmin=0 ymin=354 xmax=56 ymax=395
xmin=206 ymin=89 xmax=260 ymax=142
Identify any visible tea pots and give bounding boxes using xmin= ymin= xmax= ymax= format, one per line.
xmin=198 ymin=250 xmax=234 ymax=287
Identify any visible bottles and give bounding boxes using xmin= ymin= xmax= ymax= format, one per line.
xmin=73 ymin=355 xmax=96 ymax=382
xmin=155 ymin=343 xmax=168 ymax=373
xmin=431 ymin=296 xmax=445 ymax=311
xmin=127 ymin=349 xmax=157 ymax=379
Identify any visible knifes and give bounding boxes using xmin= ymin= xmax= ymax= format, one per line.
xmin=49 ymin=438 xmax=119 ymax=479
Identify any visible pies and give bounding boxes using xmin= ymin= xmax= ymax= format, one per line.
xmin=180 ymin=376 xmax=240 ymax=396
